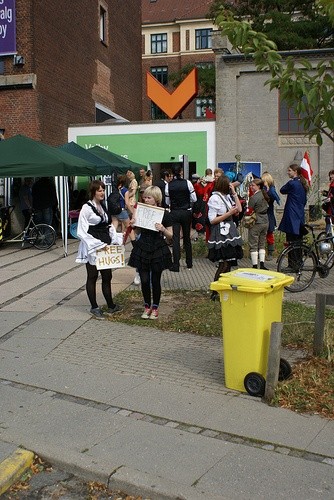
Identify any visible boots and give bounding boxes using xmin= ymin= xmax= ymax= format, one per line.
xmin=266 ymin=245 xmax=275 ymax=260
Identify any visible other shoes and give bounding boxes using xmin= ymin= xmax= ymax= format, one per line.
xmin=141 ymin=307 xmax=152 ymax=318
xmin=281 ymin=267 xmax=300 ymax=273
xmin=108 ymin=304 xmax=123 ymax=313
xmin=134 ymin=272 xmax=141 ymax=285
xmin=149 ymin=309 xmax=158 ymax=319
xmin=89 ymin=307 xmax=105 ymax=319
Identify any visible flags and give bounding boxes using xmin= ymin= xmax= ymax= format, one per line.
xmin=298 ymin=148 xmax=314 ymax=186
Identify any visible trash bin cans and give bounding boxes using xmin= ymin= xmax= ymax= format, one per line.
xmin=210 ymin=268 xmax=294 ymax=396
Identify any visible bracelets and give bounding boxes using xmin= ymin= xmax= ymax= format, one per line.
xmin=232 ymin=192 xmax=238 ymax=197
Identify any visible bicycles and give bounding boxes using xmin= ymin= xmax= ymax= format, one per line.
xmin=275 ymin=213 xmax=334 ymax=292
xmin=0 ymin=204 xmax=57 ymax=250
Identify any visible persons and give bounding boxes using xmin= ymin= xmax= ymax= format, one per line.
xmin=277 ymin=165 xmax=308 ymax=272
xmin=76 ymin=181 xmax=122 ymax=319
xmin=320 ymin=170 xmax=334 ymax=224
xmin=32 ymin=177 xmax=58 ymax=246
xmin=205 ymin=175 xmax=244 ymax=302
xmin=107 ymin=166 xmax=281 ymax=273
xmin=19 ymin=177 xmax=37 ymax=238
xmin=127 ymin=185 xmax=174 ymax=320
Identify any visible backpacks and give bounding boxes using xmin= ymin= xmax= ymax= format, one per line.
xmin=107 ymin=187 xmax=122 ymax=216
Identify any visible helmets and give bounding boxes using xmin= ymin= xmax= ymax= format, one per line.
xmin=319 ymin=242 xmax=331 ymax=254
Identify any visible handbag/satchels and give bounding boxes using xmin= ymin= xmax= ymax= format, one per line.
xmin=244 ymin=211 xmax=257 ymax=229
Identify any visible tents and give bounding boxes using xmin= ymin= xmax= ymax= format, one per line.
xmin=0 ymin=134 xmax=142 ymax=257
